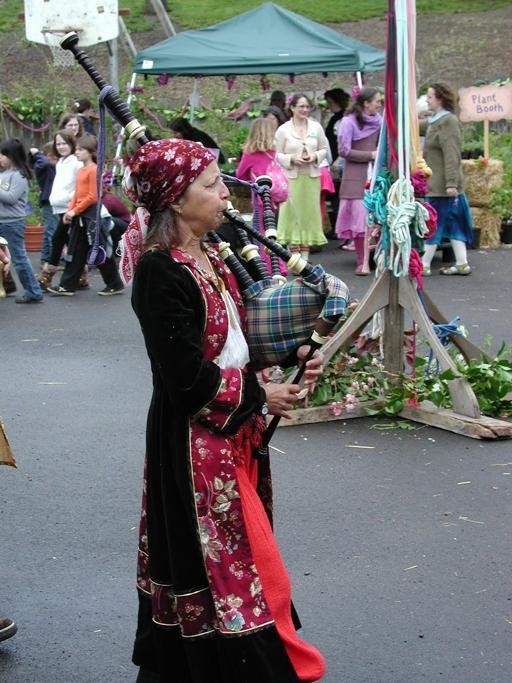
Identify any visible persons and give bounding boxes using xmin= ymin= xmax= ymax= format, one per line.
xmin=3 ymin=78 xmax=474 ymax=305
xmin=127 ymin=138 xmax=326 ymax=682
xmin=0 ymin=236 xmax=17 ymax=642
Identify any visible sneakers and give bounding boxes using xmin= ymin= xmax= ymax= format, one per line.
xmin=14 ymin=263 xmax=125 ymax=303
xmin=342 ymin=241 xmax=356 ymax=251
xmin=0 ymin=617 xmax=17 ymax=642
xmin=356 ymin=265 xmax=363 ymax=276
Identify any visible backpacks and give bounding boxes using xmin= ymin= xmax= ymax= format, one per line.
xmin=251 ymin=152 xmax=289 ymax=203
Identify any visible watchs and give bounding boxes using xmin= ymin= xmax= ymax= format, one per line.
xmin=254 ymin=387 xmax=269 ymax=416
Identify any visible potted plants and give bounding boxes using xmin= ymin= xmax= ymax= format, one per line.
xmin=23 ymin=178 xmax=44 ymax=254
xmin=490 ymin=131 xmax=512 ymax=243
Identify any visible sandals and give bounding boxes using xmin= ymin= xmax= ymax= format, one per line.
xmin=443 ymin=263 xmax=471 ymax=275
xmin=422 ymin=267 xmax=431 ymax=276
